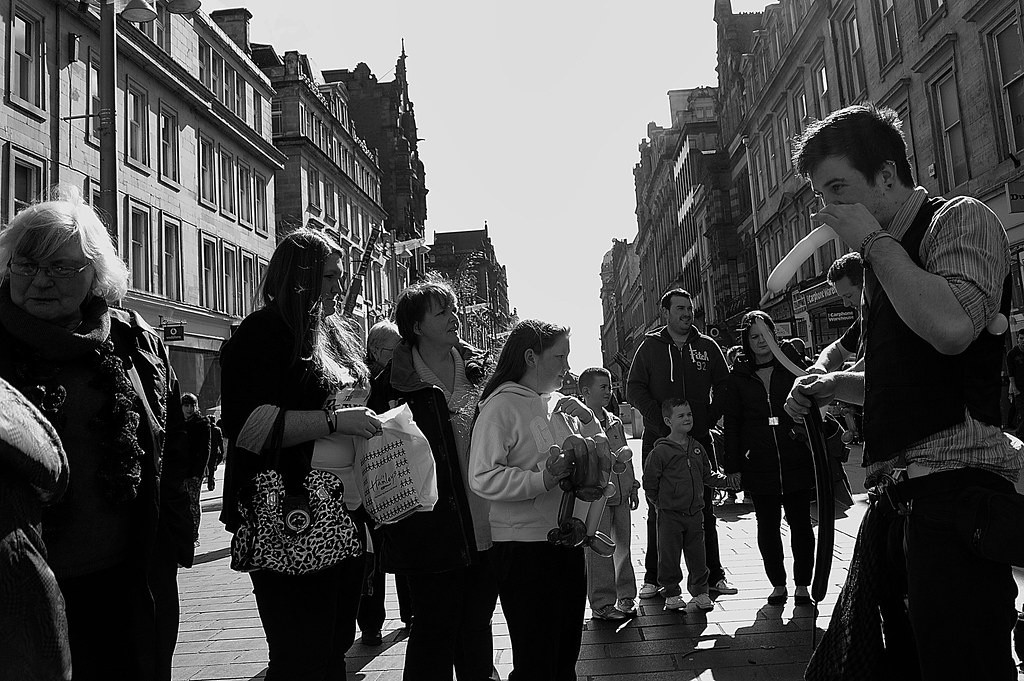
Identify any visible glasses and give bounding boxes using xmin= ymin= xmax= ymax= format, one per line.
xmin=7 ymin=249 xmax=93 ymax=277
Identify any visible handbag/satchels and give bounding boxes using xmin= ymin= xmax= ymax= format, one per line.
xmin=353 ymin=402 xmax=439 ymax=524
xmin=229 ymin=412 xmax=364 ymax=575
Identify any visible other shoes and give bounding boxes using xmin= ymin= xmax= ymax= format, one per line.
xmin=361 ymin=628 xmax=382 ymax=647
xmin=724 ymin=497 xmax=736 ymax=504
xmin=768 ymin=585 xmax=787 ymax=605
xmin=404 ymin=618 xmax=414 ymax=632
xmin=743 ymin=498 xmax=753 ymax=504
xmin=795 ymin=587 xmax=811 ymax=604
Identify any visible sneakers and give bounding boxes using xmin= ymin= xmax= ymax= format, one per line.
xmin=665 ymin=595 xmax=688 ymax=609
xmin=618 ymin=598 xmax=639 ymax=616
xmin=639 ymin=582 xmax=659 ymax=597
xmin=693 ymin=589 xmax=715 ymax=609
xmin=708 ymin=579 xmax=737 ymax=594
xmin=592 ymin=602 xmax=625 ymax=619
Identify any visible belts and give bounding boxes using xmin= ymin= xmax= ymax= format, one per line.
xmin=742 ymin=415 xmax=793 ymax=436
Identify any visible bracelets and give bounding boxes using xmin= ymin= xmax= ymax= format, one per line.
xmin=860 ymin=229 xmax=894 ymax=260
xmin=322 ymin=408 xmax=338 ymax=434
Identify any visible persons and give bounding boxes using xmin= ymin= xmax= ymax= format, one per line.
xmin=468 ymin=320 xmax=605 ymax=681
xmin=579 ymin=368 xmax=637 ymax=620
xmin=219 ymin=232 xmax=381 ymax=681
xmin=366 ymin=322 xmax=402 ymax=379
xmin=207 ymin=415 xmax=224 ymax=491
xmin=807 ymin=251 xmax=864 ymax=372
xmin=366 ymin=283 xmax=497 ymax=681
xmin=0 ymin=200 xmax=194 ymax=681
xmin=181 ymin=392 xmax=211 ymax=547
xmin=722 ymin=311 xmax=815 ymax=605
xmin=626 ymin=288 xmax=738 ymax=598
xmin=642 ymin=398 xmax=741 ymax=609
xmin=0 ymin=376 xmax=72 ymax=681
xmin=1005 ymin=329 xmax=1024 ymax=397
xmin=796 ymin=105 xmax=1024 ymax=681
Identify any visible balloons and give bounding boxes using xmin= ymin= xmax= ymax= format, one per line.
xmin=759 ymin=223 xmax=840 ymax=305
xmin=546 ymin=434 xmax=633 ymax=558
